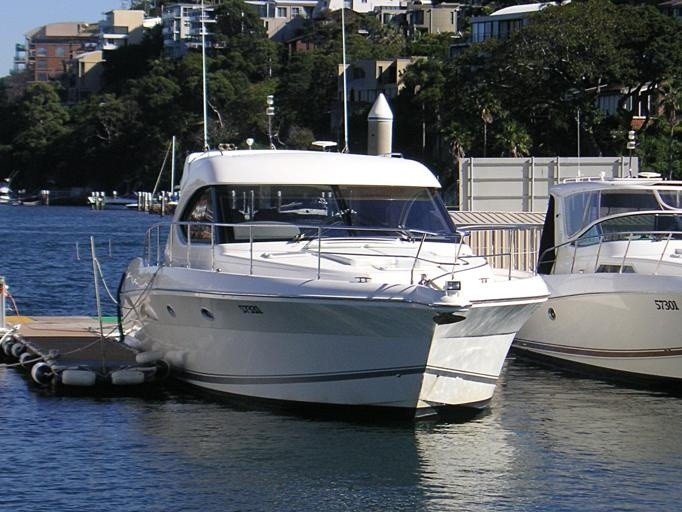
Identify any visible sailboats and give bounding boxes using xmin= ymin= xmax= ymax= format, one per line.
xmin=125 ymin=134 xmax=179 ymax=211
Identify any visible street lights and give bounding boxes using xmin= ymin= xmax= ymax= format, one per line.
xmin=245 ymin=136 xmax=255 ymax=149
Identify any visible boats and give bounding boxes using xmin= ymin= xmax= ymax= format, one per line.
xmin=113 ymin=0 xmax=552 ymax=436
xmin=510 ymin=128 xmax=682 ymax=390
xmin=0 ymin=167 xmax=61 ymax=208
